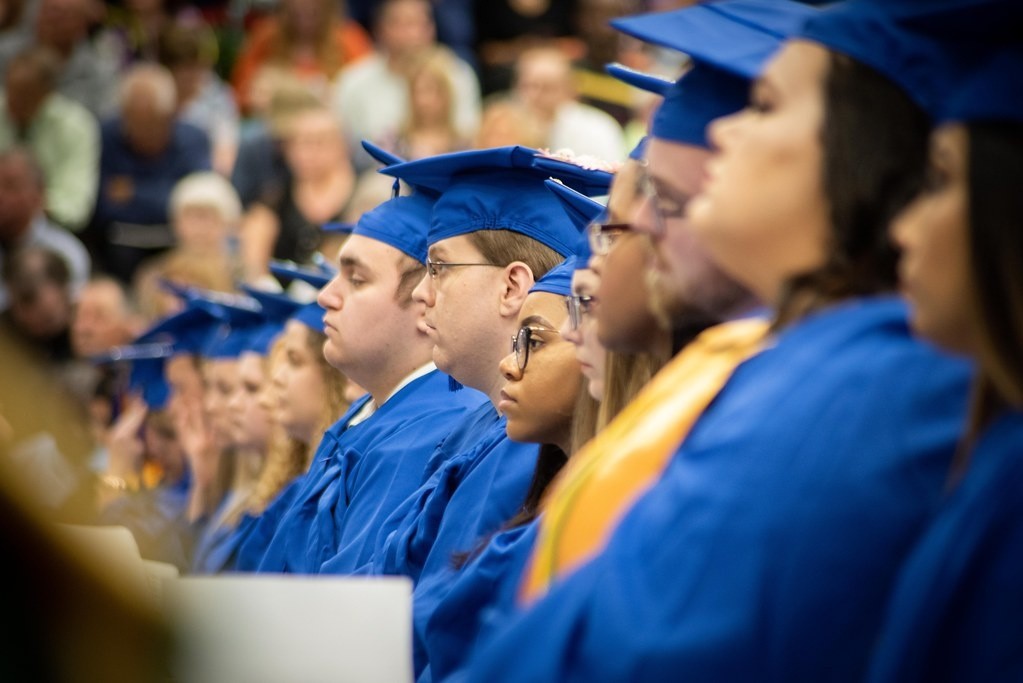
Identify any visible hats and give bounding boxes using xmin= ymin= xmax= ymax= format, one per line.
xmin=33 ymin=217 xmax=91 ymax=302
xmin=378 ymin=145 xmax=614 ymax=258
xmin=351 ymin=139 xmax=428 ymax=262
xmin=544 ymin=179 xmax=608 ymax=268
xmin=528 ymin=254 xmax=576 ymax=297
xmin=606 ymin=62 xmax=674 ymax=159
xmin=892 ymin=0 xmax=1023 ymax=122
xmin=792 ymin=0 xmax=948 ymax=110
xmin=166 ymin=172 xmax=238 ymax=223
xmin=608 ymin=0 xmax=816 ymax=152
xmin=99 ymin=257 xmax=337 ymax=411
xmin=322 ymin=224 xmax=353 ymax=233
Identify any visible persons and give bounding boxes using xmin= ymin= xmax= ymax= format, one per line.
xmin=0 ymin=0 xmax=1023 ymax=683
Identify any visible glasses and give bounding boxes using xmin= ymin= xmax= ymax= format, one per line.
xmin=564 ymin=295 xmax=600 ymax=330
xmin=512 ymin=326 xmax=562 ymax=370
xmin=588 ymin=222 xmax=636 ymax=256
xmin=426 ymin=256 xmax=538 ymax=282
